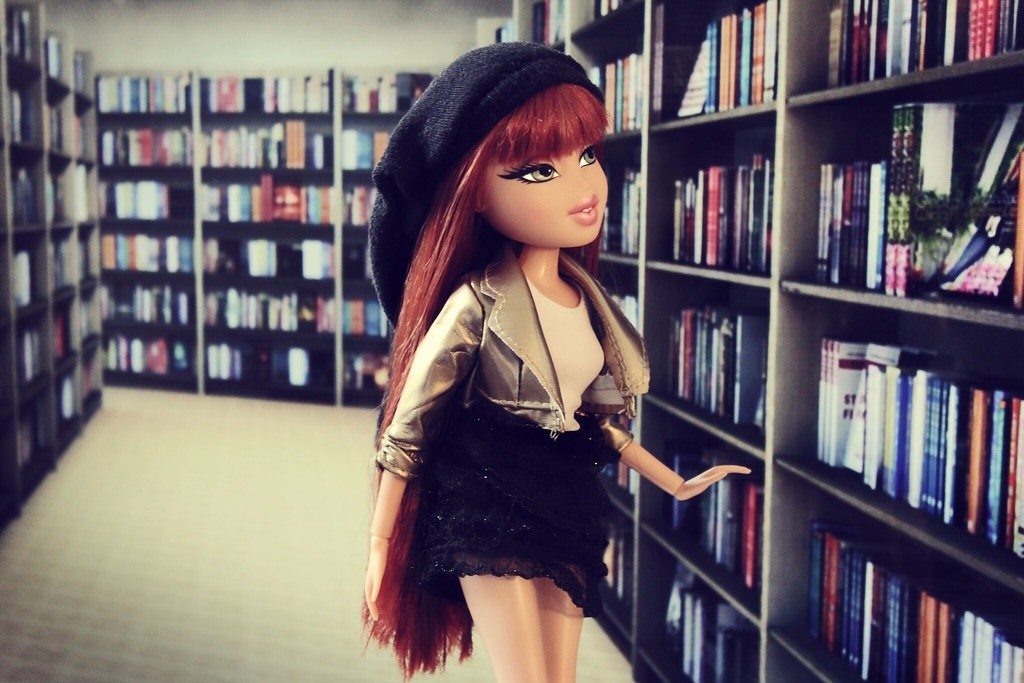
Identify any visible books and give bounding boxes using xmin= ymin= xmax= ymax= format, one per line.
xmin=0 ymin=0 xmax=1024 ymax=683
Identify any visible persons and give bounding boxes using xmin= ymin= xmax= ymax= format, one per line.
xmin=362 ymin=42 xmax=753 ymax=683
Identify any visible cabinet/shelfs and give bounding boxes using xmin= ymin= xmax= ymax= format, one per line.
xmin=0 ymin=0 xmax=104 ymax=536
xmin=104 ymin=64 xmax=434 ymax=410
xmin=508 ymin=0 xmax=1024 ymax=683
